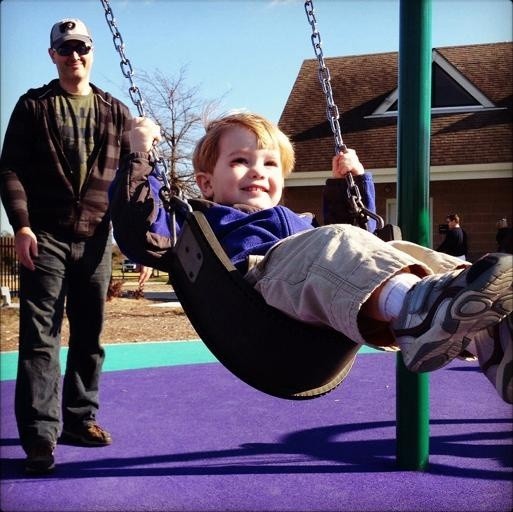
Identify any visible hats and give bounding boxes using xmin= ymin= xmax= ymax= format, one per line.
xmin=48 ymin=19 xmax=94 ymax=50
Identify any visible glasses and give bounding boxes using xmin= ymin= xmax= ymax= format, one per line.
xmin=51 ymin=44 xmax=91 ymax=57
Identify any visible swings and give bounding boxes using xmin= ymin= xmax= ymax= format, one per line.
xmin=101 ymin=0 xmax=403 ymax=402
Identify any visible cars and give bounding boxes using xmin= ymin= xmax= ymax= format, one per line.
xmin=120 ymin=259 xmax=136 ymax=273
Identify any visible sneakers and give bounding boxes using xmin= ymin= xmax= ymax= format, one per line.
xmin=21 ymin=451 xmax=56 ymax=475
xmin=472 ymin=314 xmax=512 ymax=404
xmin=389 ymin=248 xmax=512 ymax=375
xmin=60 ymin=421 xmax=112 ymax=446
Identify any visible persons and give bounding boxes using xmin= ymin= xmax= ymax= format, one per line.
xmin=0 ymin=18 xmax=154 ymax=476
xmin=435 ymin=210 xmax=469 ymax=263
xmin=493 ymin=214 xmax=513 ymax=255
xmin=105 ymin=111 xmax=511 ymax=407
